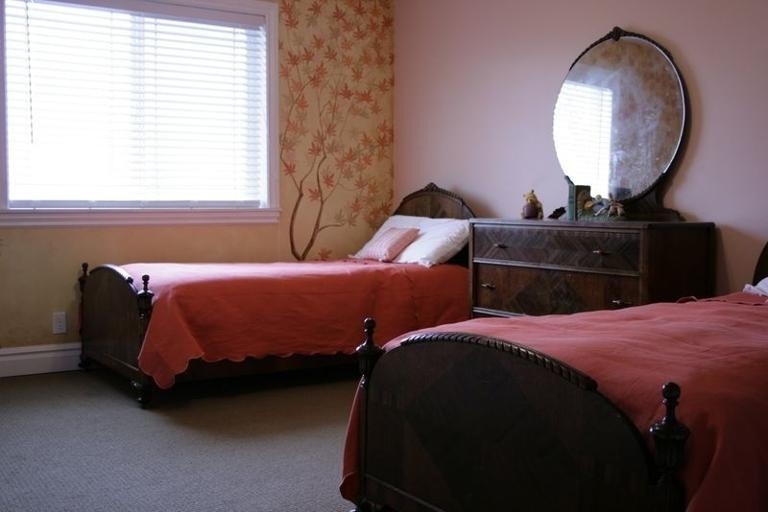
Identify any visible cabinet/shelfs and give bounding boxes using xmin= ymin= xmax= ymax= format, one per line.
xmin=468 ymin=218 xmax=715 ymax=323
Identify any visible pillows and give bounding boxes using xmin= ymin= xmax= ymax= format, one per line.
xmin=357 ymin=228 xmax=419 ymax=261
xmin=353 ymin=215 xmax=469 ymax=268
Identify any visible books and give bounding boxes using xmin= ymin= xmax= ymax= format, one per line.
xmin=568 ymin=183 xmax=591 ymax=222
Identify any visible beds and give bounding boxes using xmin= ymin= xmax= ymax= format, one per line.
xmin=353 ymin=242 xmax=767 ymax=512
xmin=78 ymin=182 xmax=475 ymax=411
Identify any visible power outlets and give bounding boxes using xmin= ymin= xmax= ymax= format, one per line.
xmin=53 ymin=312 xmax=67 ymax=335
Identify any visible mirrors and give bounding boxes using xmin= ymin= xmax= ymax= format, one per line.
xmin=552 ymin=25 xmax=690 ymax=222
